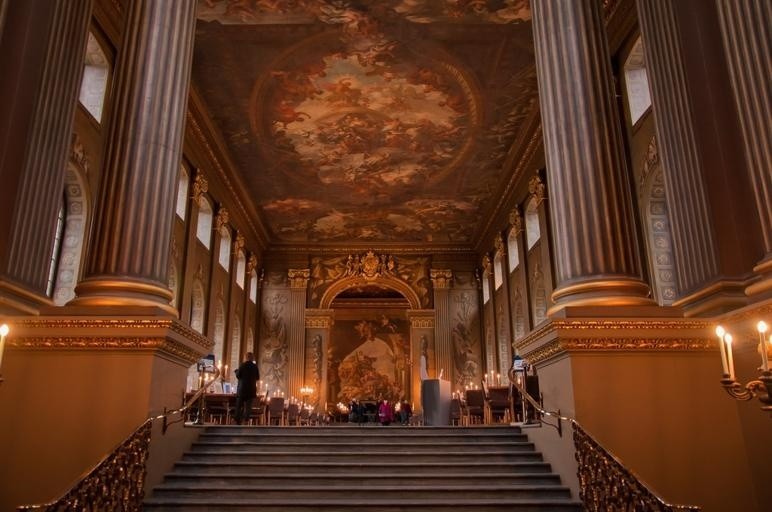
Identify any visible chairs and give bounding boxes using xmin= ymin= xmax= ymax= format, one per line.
xmin=408 ymin=376 xmax=539 ymax=427
xmin=185 ymin=386 xmax=331 ymax=426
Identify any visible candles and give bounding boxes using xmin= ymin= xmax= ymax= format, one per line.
xmin=715 ymin=325 xmax=727 ymax=375
xmin=496 ymin=373 xmax=500 ymax=385
xmin=725 ymin=334 xmax=735 ymax=381
xmin=0 ymin=323 xmax=9 ymax=366
xmin=756 ymin=319 xmax=769 ymax=372
xmin=215 ymin=359 xmax=269 ymax=396
xmin=484 ymin=372 xmax=487 ymax=385
xmin=491 ymin=369 xmax=494 ymax=386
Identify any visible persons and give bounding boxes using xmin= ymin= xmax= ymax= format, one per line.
xmin=234 ymin=352 xmax=260 ymax=425
xmin=336 ymin=399 xmax=411 ymax=426
